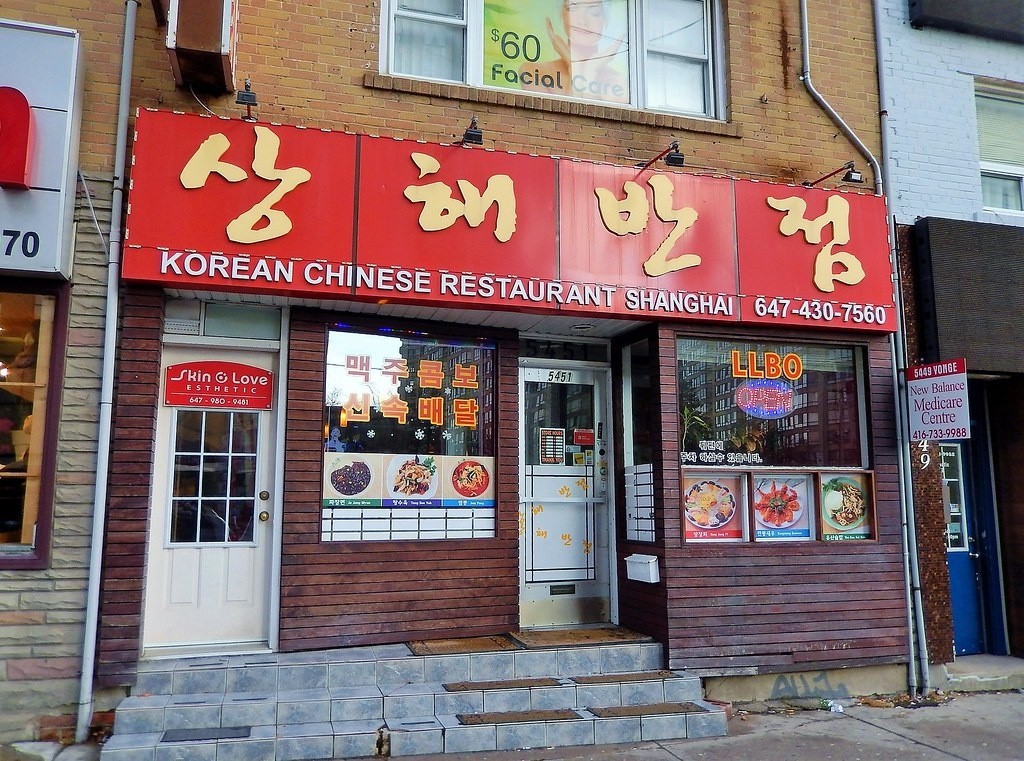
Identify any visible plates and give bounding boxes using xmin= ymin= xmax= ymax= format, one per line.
xmin=821 ymin=477 xmax=867 ymax=531
xmin=755 ymin=483 xmax=803 ymax=529
xmin=448 ymin=458 xmax=493 ymax=499
xmin=325 ymin=454 xmax=374 ymax=496
xmin=685 ymin=479 xmax=736 ymax=529
xmin=386 ymin=455 xmax=438 ymax=499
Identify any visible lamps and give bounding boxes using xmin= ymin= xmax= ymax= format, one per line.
xmin=235 ymin=78 xmax=258 ymax=118
xmin=805 ymin=161 xmax=865 ymax=187
xmin=460 ymin=115 xmax=484 ymax=145
xmin=642 ymin=141 xmax=684 ymax=169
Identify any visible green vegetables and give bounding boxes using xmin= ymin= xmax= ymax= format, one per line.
xmin=422 ymin=456 xmax=437 ymax=476
xmin=822 ymin=478 xmax=848 ymax=493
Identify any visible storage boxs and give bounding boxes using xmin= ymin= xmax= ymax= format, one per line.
xmin=624 ymin=554 xmax=661 ymax=584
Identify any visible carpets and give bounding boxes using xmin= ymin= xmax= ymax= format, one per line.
xmin=160 ymin=725 xmax=250 ymax=742
xmin=454 ymin=707 xmax=584 ymax=725
xmin=505 ymin=626 xmax=658 ymax=650
xmin=586 ymin=701 xmax=710 ymax=718
xmin=567 ymin=670 xmax=684 ymax=684
xmin=404 ymin=634 xmax=525 ymax=656
xmin=442 ymin=677 xmax=563 ymax=692
